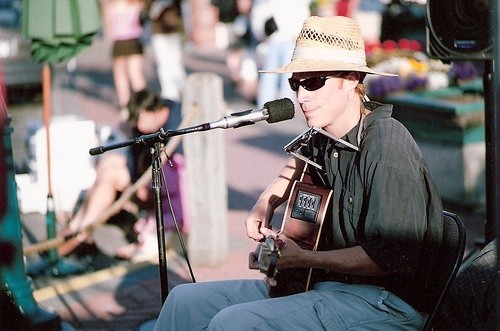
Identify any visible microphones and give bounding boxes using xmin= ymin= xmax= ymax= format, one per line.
xmin=166 ymin=96 xmax=295 ymax=137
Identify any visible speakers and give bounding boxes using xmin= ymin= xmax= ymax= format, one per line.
xmin=427 ymin=0 xmax=495 ymax=64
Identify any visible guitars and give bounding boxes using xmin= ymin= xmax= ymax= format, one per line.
xmin=256 ymin=180 xmax=333 ymax=298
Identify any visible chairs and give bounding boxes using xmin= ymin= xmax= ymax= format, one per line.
xmin=424 ymin=210 xmax=467 ymax=331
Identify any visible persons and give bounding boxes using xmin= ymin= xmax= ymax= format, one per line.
xmin=153 ymin=16 xmax=444 ymax=331
xmin=57 ymin=0 xmax=311 ymax=263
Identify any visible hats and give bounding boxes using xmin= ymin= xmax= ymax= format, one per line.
xmin=258 ymin=15 xmax=399 ymax=77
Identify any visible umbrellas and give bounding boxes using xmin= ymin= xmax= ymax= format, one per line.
xmin=23 ymin=0 xmax=105 ymax=195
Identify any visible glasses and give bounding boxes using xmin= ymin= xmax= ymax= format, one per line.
xmin=288 ymin=72 xmax=343 ymax=92
